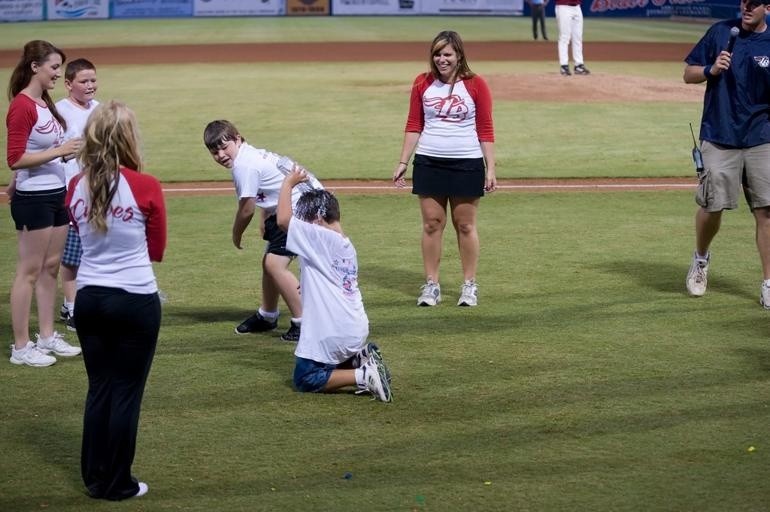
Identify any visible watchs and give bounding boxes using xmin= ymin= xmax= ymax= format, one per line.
xmin=703 ymin=64 xmax=710 ymax=81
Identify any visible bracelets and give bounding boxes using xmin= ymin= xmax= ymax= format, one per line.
xmin=399 ymin=159 xmax=410 ymax=166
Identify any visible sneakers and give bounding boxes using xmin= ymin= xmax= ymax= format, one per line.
xmin=355 ymin=344 xmax=381 ymax=364
xmin=686 ymin=252 xmax=709 ymax=299
xmin=758 ymin=283 xmax=769 ymax=310
xmin=35 ymin=331 xmax=83 ymax=358
xmin=139 ymin=482 xmax=150 ymax=491
xmin=282 ymin=319 xmax=301 ymax=340
xmin=417 ymin=281 xmax=442 ymax=306
xmin=457 ymin=284 xmax=480 ymax=308
xmin=561 ymin=65 xmax=571 ymax=73
xmin=235 ymin=310 xmax=281 ymax=335
xmin=574 ymin=65 xmax=588 ymax=75
xmin=61 ymin=306 xmax=77 ymax=331
xmin=9 ymin=342 xmax=57 ymax=368
xmin=362 ymin=353 xmax=394 ymax=403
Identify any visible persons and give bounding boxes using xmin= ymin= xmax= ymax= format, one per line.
xmin=64 ymin=101 xmax=167 ymax=501
xmin=8 ymin=39 xmax=82 ymax=367
xmin=4 ymin=58 xmax=103 ymax=333
xmin=684 ymin=0 xmax=769 ymax=309
xmin=555 ymin=0 xmax=590 ymax=76
xmin=204 ymin=119 xmax=323 ymax=342
xmin=529 ymin=1 xmax=548 ymax=41
xmin=392 ymin=29 xmax=497 ymax=305
xmin=275 ymin=161 xmax=394 ymax=402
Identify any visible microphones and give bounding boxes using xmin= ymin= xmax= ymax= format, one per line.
xmin=727 ymin=26 xmax=740 ymax=54
